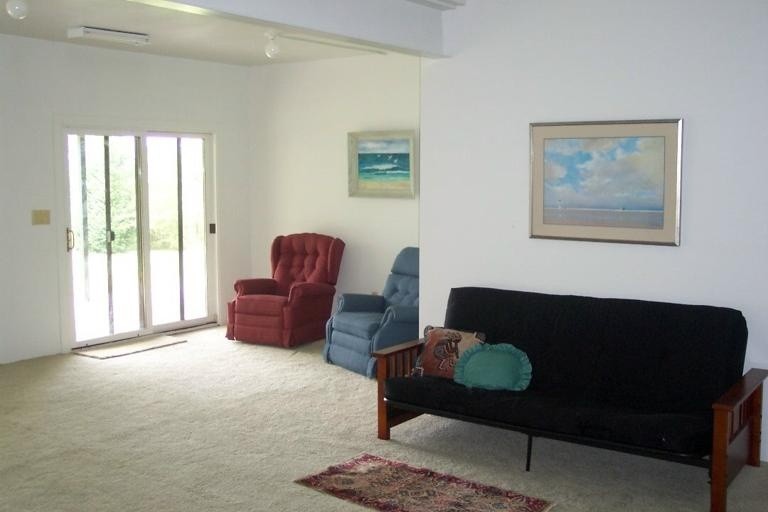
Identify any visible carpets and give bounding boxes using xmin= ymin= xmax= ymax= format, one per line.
xmin=72 ymin=332 xmax=187 ymax=361
xmin=293 ymin=454 xmax=554 ymax=511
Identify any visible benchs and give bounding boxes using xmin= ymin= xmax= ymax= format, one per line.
xmin=373 ymin=285 xmax=768 ymax=511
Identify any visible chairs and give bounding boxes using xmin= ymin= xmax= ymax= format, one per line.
xmin=224 ymin=231 xmax=419 ymax=378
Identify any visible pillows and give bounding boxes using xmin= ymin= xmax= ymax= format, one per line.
xmin=410 ymin=323 xmax=535 ymax=392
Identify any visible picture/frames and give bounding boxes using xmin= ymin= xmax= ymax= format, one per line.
xmin=527 ymin=117 xmax=683 ymax=250
xmin=345 ymin=128 xmax=419 ymax=201
